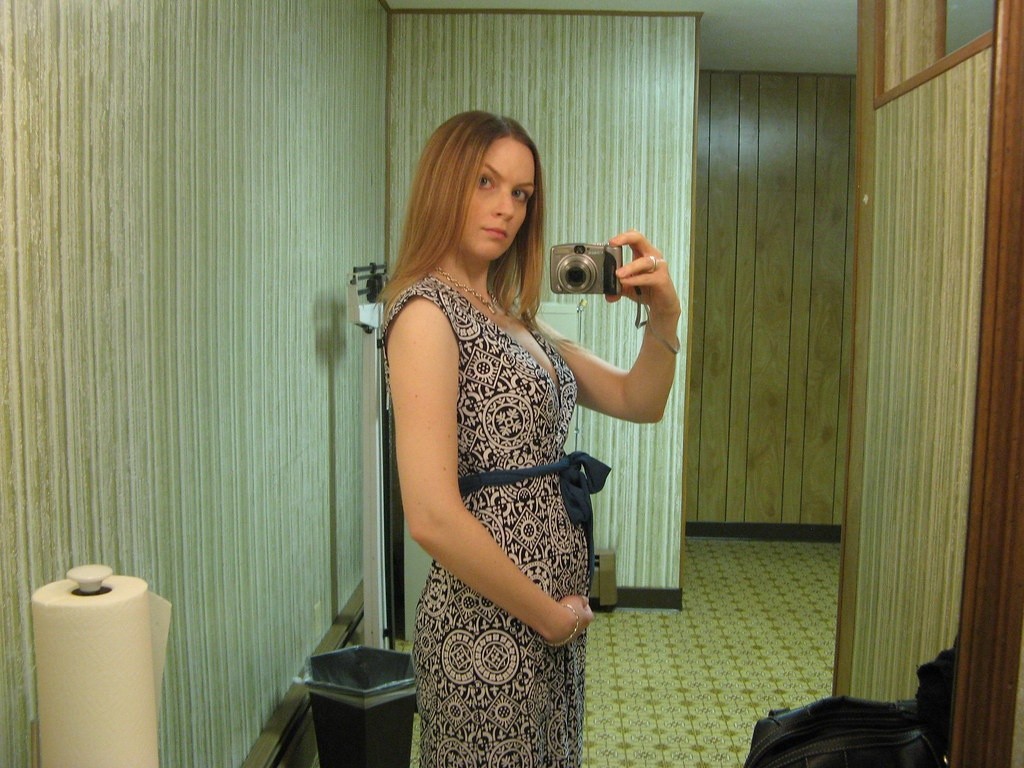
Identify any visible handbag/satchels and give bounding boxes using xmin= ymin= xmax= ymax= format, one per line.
xmin=743 ymin=695 xmax=949 ymax=768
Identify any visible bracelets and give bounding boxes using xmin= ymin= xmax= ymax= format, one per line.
xmin=544 ymin=604 xmax=580 ymax=648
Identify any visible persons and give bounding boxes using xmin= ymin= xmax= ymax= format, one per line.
xmin=379 ymin=110 xmax=681 ymax=768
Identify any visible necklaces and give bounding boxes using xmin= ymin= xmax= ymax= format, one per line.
xmin=433 ymin=262 xmax=499 ymax=314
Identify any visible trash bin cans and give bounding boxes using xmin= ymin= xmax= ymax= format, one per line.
xmin=307 ymin=646 xmax=416 ymax=768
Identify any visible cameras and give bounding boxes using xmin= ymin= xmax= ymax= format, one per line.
xmin=550 ymin=244 xmax=624 ymax=296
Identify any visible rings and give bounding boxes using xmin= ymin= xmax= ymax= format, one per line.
xmin=649 ymin=256 xmax=657 ymax=274
